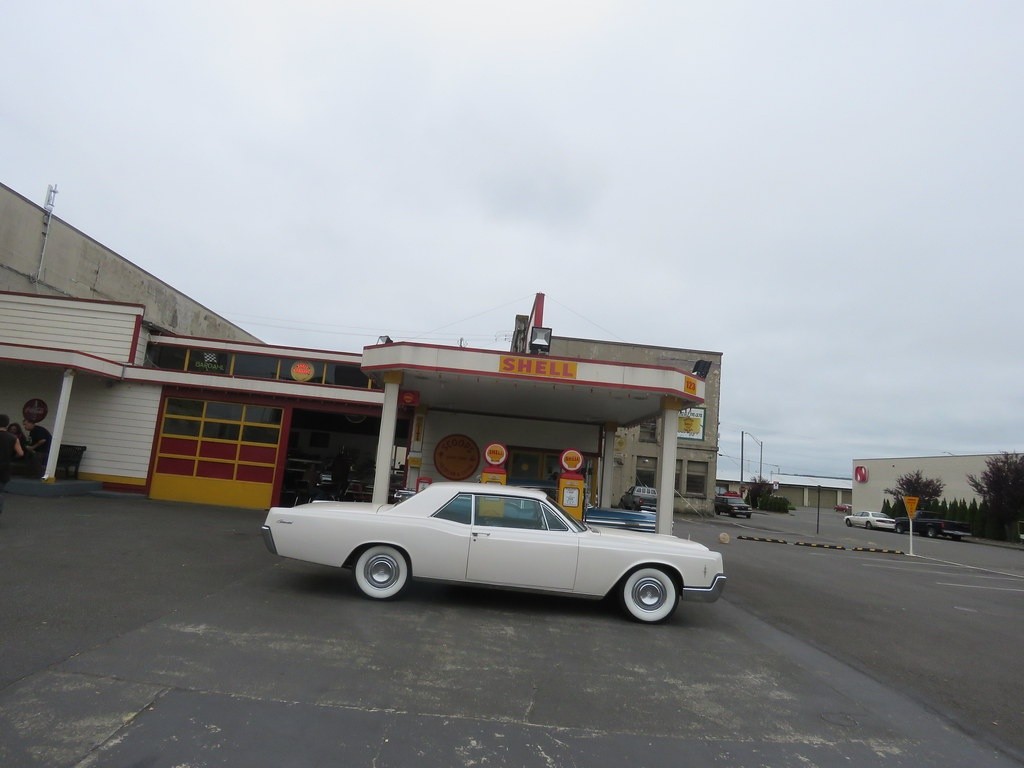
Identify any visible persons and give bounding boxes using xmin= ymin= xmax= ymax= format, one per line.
xmin=0 ymin=414 xmax=52 ymax=489
xmin=325 ymin=446 xmax=349 ymax=501
xmin=302 ymin=462 xmax=325 ymax=501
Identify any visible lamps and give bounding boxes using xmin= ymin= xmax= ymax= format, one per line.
xmin=377 ymin=336 xmax=393 ymax=345
xmin=692 ymin=359 xmax=713 ymax=379
xmin=530 ymin=327 xmax=552 ymax=355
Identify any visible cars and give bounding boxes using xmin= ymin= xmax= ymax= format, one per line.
xmin=845 ymin=511 xmax=895 ymax=531
xmin=262 ymin=483 xmax=728 ymax=623
xmin=834 ymin=503 xmax=850 ymax=511
xmin=393 ymin=488 xmax=674 ymax=537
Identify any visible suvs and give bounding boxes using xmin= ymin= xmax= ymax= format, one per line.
xmin=713 ymin=495 xmax=752 ymax=519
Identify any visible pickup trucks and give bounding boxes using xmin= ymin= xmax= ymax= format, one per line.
xmin=895 ymin=509 xmax=973 ymax=540
xmin=620 ymin=486 xmax=657 ymax=510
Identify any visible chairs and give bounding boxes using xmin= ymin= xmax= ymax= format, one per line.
xmin=282 ymin=472 xmax=312 ymax=507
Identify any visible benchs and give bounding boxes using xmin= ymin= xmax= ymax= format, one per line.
xmin=38 ymin=445 xmax=88 ymax=477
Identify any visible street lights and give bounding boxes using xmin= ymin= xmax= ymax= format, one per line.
xmin=745 ymin=432 xmax=762 ymax=484
